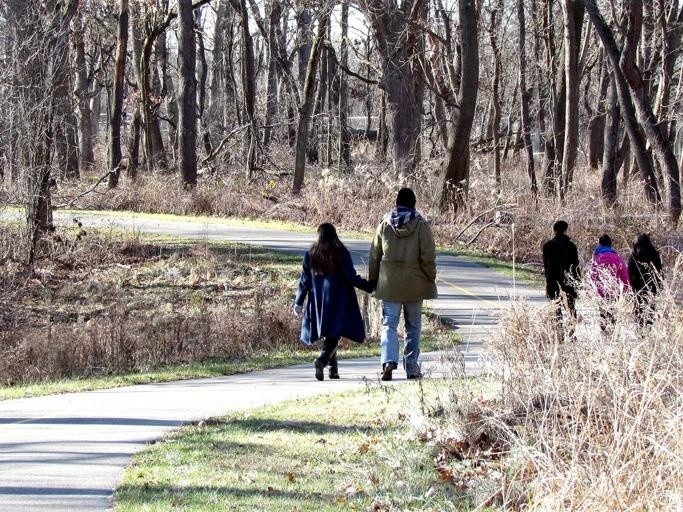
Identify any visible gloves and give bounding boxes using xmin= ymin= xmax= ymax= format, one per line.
xmin=293 ymin=304 xmax=302 ymax=316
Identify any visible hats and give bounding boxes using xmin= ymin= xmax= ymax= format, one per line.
xmin=396 ymin=188 xmax=416 ymax=208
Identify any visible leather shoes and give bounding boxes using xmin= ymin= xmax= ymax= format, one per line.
xmin=407 ymin=372 xmax=423 ymax=379
xmin=381 ymin=362 xmax=392 ymax=381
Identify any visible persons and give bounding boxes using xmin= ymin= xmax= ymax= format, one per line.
xmin=589 ymin=235 xmax=630 ymax=337
xmin=369 ymin=187 xmax=437 ymax=379
xmin=544 ymin=220 xmax=580 ymax=342
xmin=628 ymin=234 xmax=663 ymax=337
xmin=292 ymin=222 xmax=374 ymax=380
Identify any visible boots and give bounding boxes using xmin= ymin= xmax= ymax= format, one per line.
xmin=314 ymin=351 xmax=340 ymax=381
